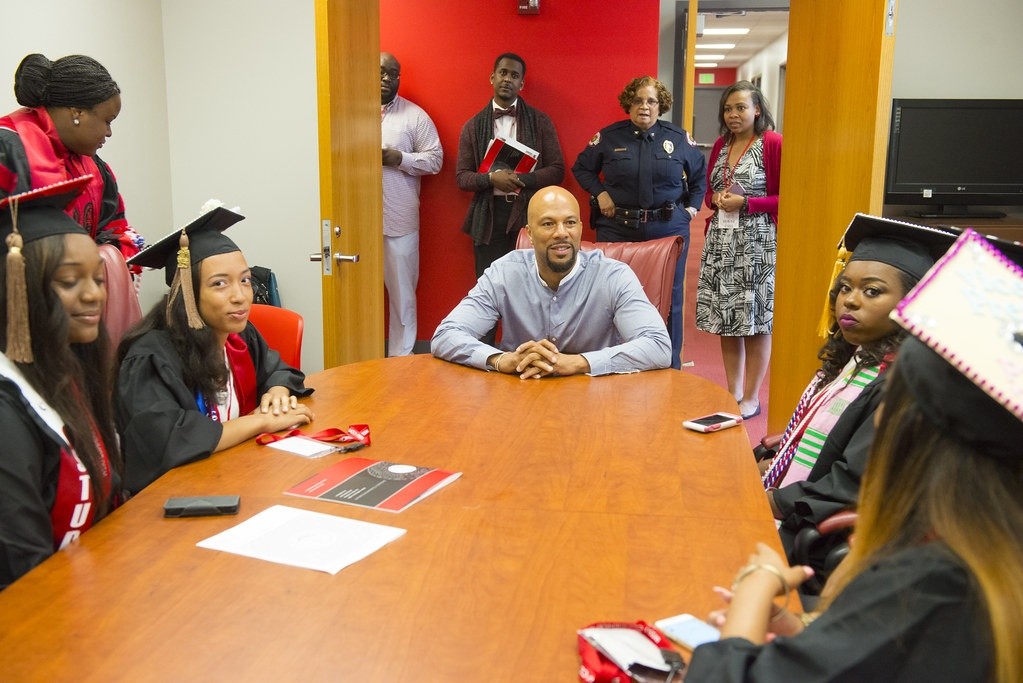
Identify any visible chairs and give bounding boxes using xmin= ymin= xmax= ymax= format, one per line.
xmin=514 ymin=227 xmax=684 ymax=324
xmin=245 ymin=305 xmax=305 ymax=374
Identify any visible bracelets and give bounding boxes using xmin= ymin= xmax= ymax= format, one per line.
xmin=495 ymin=351 xmax=511 ymax=372
xmin=742 ymin=195 xmax=747 ymax=211
xmin=732 ymin=564 xmax=790 ymax=624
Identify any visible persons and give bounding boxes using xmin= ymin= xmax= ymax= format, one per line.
xmin=430 ymin=186 xmax=672 ymax=380
xmin=0 ymin=54 xmax=143 ymax=294
xmin=571 ymin=75 xmax=706 ymax=370
xmin=704 ymin=81 xmax=782 ymax=420
xmin=759 ymin=212 xmax=961 ymax=558
xmin=456 ymin=52 xmax=566 ymax=348
xmin=0 ymin=172 xmax=121 ymax=593
xmin=112 ymin=208 xmax=316 ymax=501
xmin=684 ymin=231 xmax=1023 ymax=683
xmin=381 ymin=52 xmax=443 ymax=357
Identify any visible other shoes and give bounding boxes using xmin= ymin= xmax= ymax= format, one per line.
xmin=735 ymin=394 xmax=761 ymax=419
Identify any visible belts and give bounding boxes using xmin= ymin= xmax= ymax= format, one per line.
xmin=615 ymin=194 xmax=685 ymax=223
xmin=494 ymin=195 xmax=518 ymax=203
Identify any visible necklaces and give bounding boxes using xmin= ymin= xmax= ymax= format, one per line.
xmin=761 ymin=371 xmax=839 ymax=489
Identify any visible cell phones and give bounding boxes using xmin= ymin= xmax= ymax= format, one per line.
xmin=683 ymin=412 xmax=742 ymax=433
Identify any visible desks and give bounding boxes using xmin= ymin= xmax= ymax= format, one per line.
xmin=0 ymin=355 xmax=800 ymax=683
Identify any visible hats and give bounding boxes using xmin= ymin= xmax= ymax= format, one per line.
xmin=0 ymin=175 xmax=95 ymax=364
xmin=125 ymin=207 xmax=246 ymax=330
xmin=889 ymin=229 xmax=1023 ymax=467
xmin=936 ymin=224 xmax=1023 ymax=267
xmin=818 ymin=213 xmax=957 ymax=338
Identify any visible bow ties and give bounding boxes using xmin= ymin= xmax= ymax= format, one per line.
xmin=494 ymin=105 xmax=515 ymax=119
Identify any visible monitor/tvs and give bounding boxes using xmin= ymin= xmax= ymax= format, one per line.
xmin=885 ymin=99 xmax=1023 ymax=219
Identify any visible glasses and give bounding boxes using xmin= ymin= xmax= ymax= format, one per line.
xmin=381 ymin=69 xmax=400 ymax=80
xmin=631 ymin=97 xmax=659 ymax=108
